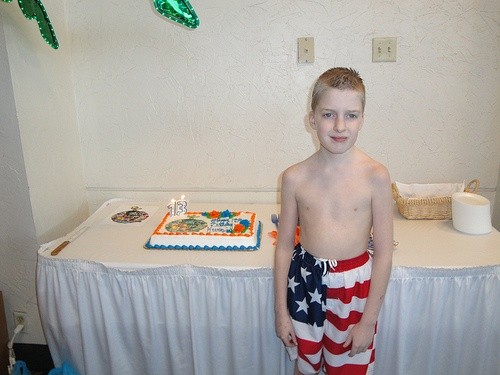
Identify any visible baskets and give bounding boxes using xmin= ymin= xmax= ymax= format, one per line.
xmin=392 ymin=178 xmax=479 ymax=222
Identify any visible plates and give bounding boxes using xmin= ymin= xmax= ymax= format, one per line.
xmin=452 ymin=192 xmax=491 ymax=234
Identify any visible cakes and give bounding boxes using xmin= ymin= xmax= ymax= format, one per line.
xmin=145 ymin=209 xmax=262 ymax=250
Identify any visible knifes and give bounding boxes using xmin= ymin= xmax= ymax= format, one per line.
xmin=51 ymin=226 xmax=88 ymax=256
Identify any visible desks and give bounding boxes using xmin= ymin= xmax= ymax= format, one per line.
xmin=36 ymin=198 xmax=500 ymax=374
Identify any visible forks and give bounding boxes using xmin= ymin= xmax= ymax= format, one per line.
xmin=270 ymin=213 xmax=278 ymax=229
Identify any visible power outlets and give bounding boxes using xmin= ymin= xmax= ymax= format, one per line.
xmin=13 ymin=311 xmax=30 ymax=334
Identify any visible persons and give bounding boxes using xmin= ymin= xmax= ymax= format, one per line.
xmin=273 ymin=67 xmax=394 ymax=375
